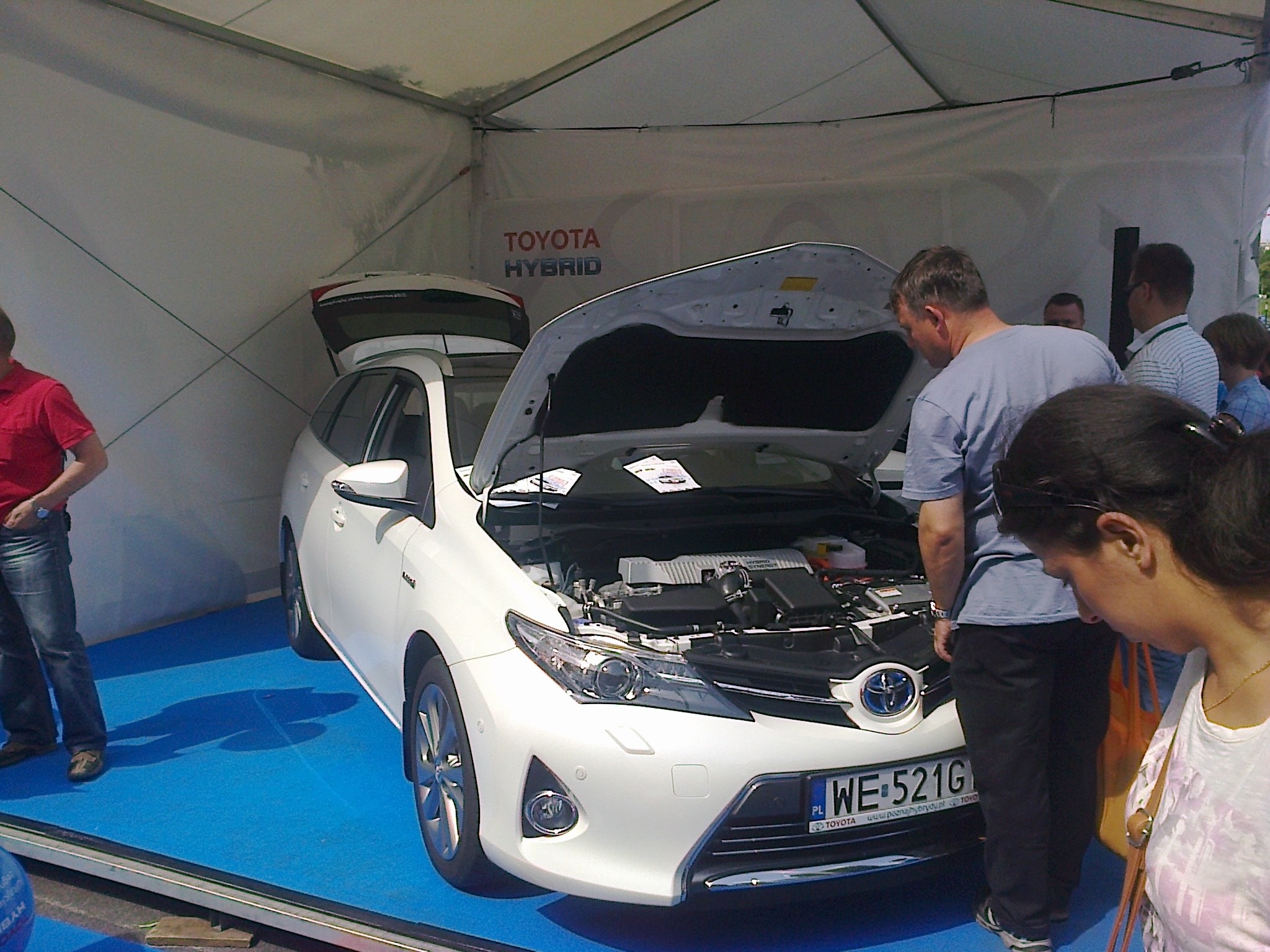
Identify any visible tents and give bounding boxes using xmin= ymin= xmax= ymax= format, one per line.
xmin=0 ymin=0 xmax=1270 ymax=650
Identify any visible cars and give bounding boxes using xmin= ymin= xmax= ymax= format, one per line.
xmin=275 ymin=242 xmax=991 ymax=909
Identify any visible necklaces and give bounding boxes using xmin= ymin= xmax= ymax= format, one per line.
xmin=1204 ymin=661 xmax=1270 ymax=711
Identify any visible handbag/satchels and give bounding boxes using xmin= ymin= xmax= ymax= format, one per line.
xmin=1090 ymin=636 xmax=1163 ymax=862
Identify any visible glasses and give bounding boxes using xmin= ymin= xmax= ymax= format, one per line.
xmin=991 ymin=460 xmax=1113 ymax=519
xmin=1124 ymin=282 xmax=1142 ymax=303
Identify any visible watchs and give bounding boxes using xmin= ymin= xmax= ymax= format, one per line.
xmin=930 ymin=602 xmax=951 ymax=618
xmin=29 ymin=497 xmax=49 ymax=519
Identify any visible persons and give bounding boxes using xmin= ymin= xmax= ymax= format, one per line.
xmin=1203 ymin=312 xmax=1270 ymax=434
xmin=1259 ymin=331 xmax=1270 ymax=390
xmin=1123 ymin=243 xmax=1219 ymax=717
xmin=0 ymin=308 xmax=107 ymax=782
xmin=891 ymin=246 xmax=1123 ymax=952
xmin=1043 ymin=292 xmax=1085 ymax=329
xmin=994 ymin=382 xmax=1270 ymax=952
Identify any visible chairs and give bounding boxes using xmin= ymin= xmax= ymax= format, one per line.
xmin=389 ymin=414 xmax=427 ymax=458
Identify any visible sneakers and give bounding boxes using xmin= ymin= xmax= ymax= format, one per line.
xmin=0 ymin=737 xmax=57 ymax=767
xmin=67 ymin=750 xmax=103 ymax=780
xmin=1052 ymin=907 xmax=1067 ymax=922
xmin=970 ymin=888 xmax=1053 ymax=952
